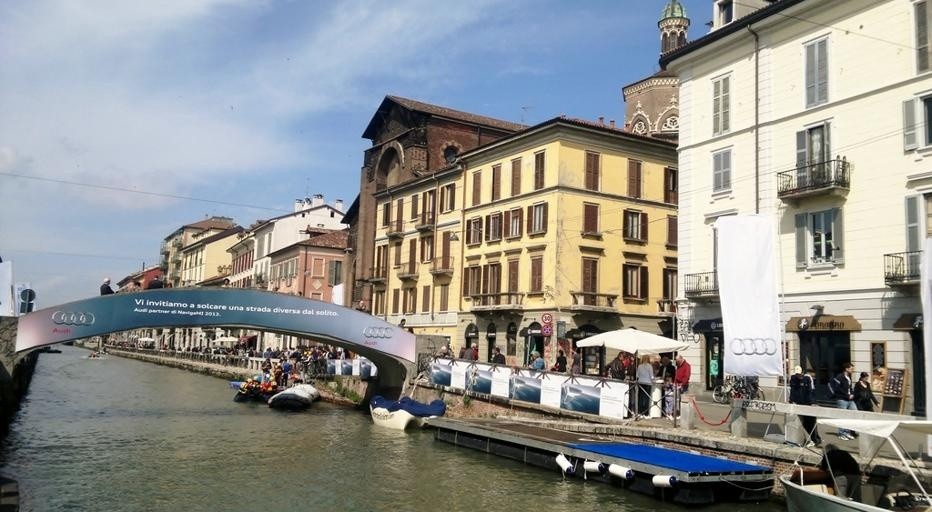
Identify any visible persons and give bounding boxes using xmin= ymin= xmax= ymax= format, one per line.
xmin=355 ymin=300 xmax=367 ymax=312
xmin=407 ymin=327 xmax=413 ymax=334
xmin=786 ymin=363 xmax=882 ymax=447
xmin=221 ymin=278 xmax=232 ymax=288
xmin=398 ymin=319 xmax=406 ymax=328
xmin=817 ymin=442 xmax=861 ymax=499
xmin=89 ymin=335 xmax=356 ymax=399
xmin=166 ymin=283 xmax=172 ymax=288
xmin=435 ymin=339 xmax=691 ymax=418
xmin=100 ymin=278 xmax=114 ymax=296
xmin=129 ymin=281 xmax=144 ymax=292
xmin=149 ymin=274 xmax=164 ymax=289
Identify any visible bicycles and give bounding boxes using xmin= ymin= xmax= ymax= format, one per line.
xmin=712 ymin=375 xmax=766 ymax=405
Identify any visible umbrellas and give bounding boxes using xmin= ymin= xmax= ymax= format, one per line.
xmin=575 ymin=327 xmax=690 ymax=382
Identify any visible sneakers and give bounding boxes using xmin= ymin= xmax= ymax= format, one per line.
xmin=666 ymin=414 xmax=673 ymax=419
xmin=838 ymin=432 xmax=859 ymax=440
xmin=639 ymin=414 xmax=650 ymax=419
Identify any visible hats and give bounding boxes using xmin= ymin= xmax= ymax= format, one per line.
xmin=794 ymin=365 xmax=802 ymax=374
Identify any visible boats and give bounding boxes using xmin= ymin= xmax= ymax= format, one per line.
xmin=778 ymin=418 xmax=932 ymax=511
xmin=229 ymin=380 xmax=283 ymax=401
xmin=369 ymin=395 xmax=446 ymax=431
